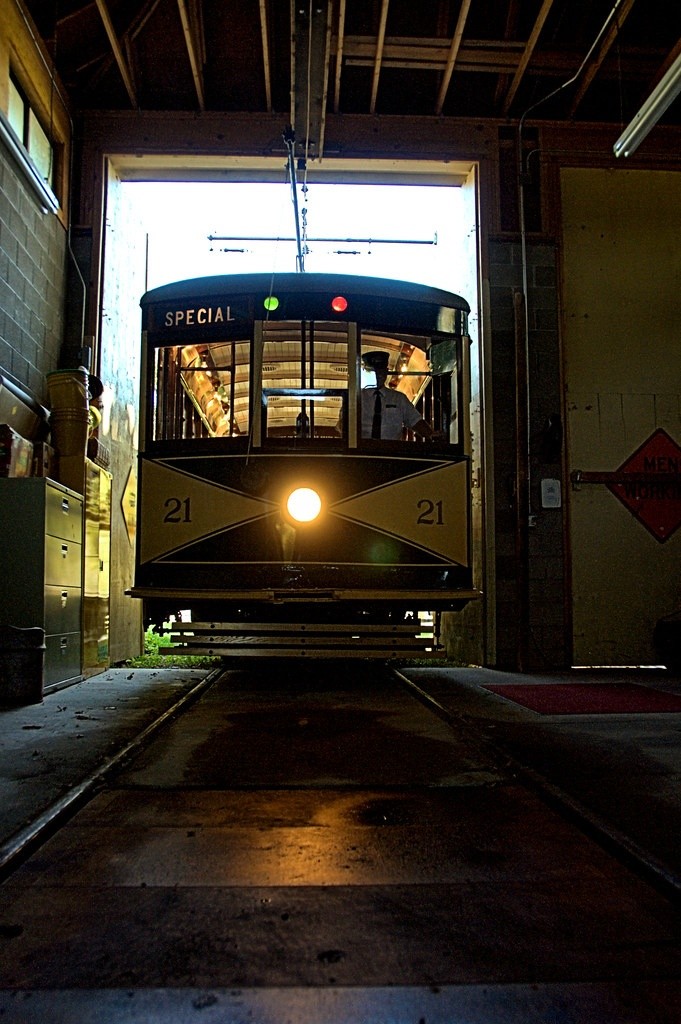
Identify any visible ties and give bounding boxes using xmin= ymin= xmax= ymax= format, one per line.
xmin=369 ymin=390 xmax=383 ymax=440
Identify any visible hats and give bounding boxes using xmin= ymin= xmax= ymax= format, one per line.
xmin=361 ymin=351 xmax=389 ymax=370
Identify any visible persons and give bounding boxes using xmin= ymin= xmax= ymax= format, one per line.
xmin=335 ymin=351 xmax=445 ymax=443
xmin=86 ymin=374 xmax=106 ymax=440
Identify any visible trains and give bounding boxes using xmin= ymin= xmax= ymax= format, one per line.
xmin=137 ymin=271 xmax=490 ymax=676
